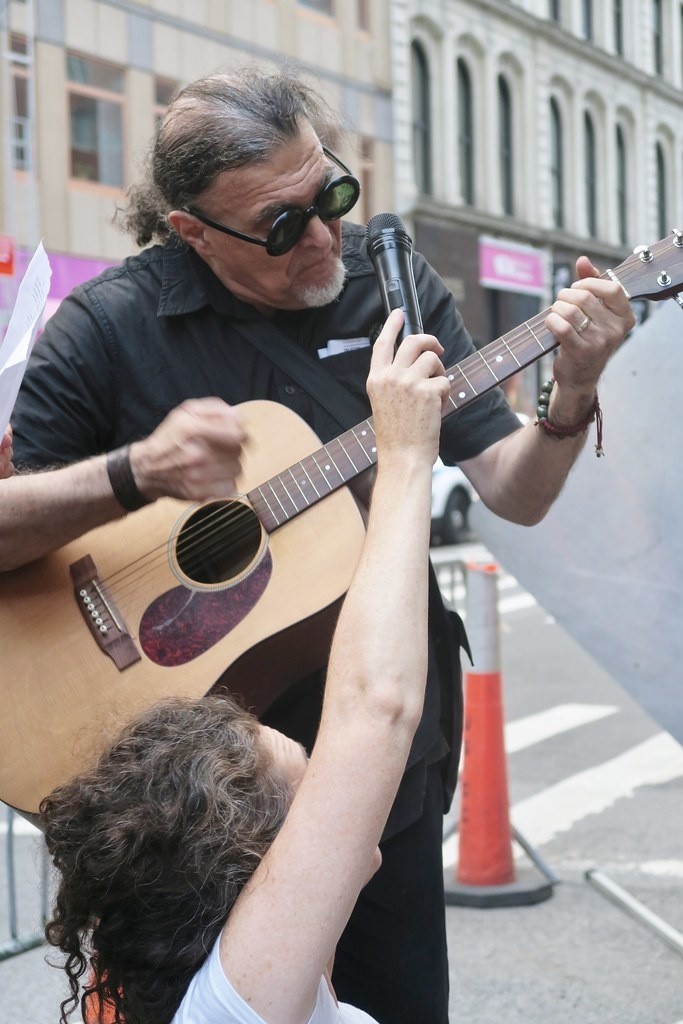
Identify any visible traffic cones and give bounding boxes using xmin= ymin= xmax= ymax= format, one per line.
xmin=431 ymin=562 xmax=553 ymax=911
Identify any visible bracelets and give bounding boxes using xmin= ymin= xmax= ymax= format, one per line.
xmin=106 ymin=444 xmax=153 ymax=512
xmin=535 ymin=379 xmax=605 ymax=458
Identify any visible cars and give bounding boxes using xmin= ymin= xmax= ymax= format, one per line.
xmin=431 ymin=410 xmax=534 ymax=548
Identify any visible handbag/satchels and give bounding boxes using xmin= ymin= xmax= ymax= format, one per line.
xmin=439 ymin=610 xmax=474 ymax=814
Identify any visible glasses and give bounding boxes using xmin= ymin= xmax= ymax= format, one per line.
xmin=182 ymin=144 xmax=360 ymax=257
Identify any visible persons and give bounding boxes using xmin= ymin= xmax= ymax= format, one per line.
xmin=1 ymin=65 xmax=635 ymax=1023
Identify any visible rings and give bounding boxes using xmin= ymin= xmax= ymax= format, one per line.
xmin=577 ymin=316 xmax=590 ymax=335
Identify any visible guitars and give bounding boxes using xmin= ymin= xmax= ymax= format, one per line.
xmin=2 ymin=227 xmax=683 ymax=833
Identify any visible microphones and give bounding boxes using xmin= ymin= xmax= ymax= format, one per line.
xmin=365 ymin=213 xmax=423 ymax=350
